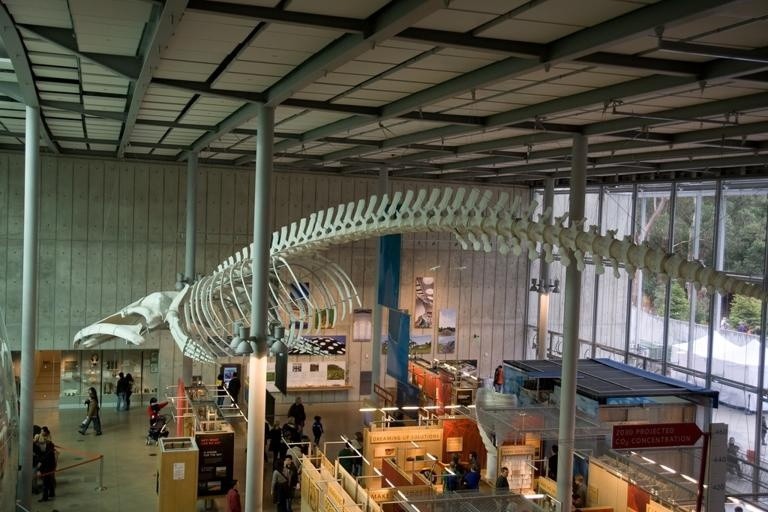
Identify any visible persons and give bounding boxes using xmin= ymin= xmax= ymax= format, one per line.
xmin=124 ymin=373 xmax=134 ymax=411
xmin=493 ymin=365 xmax=504 ymax=393
xmin=339 ymin=440 xmax=356 ymax=475
xmin=227 ymin=480 xmax=241 ymax=512
xmin=227 ymin=372 xmax=241 ymax=407
xmin=575 ymin=474 xmax=587 ymax=508
xmin=351 ymin=432 xmax=363 ymax=476
xmin=312 ymin=416 xmax=324 ymax=445
xmin=115 ymin=372 xmax=127 ymax=411
xmin=447 ymin=452 xmax=481 ymax=492
xmin=496 ymin=467 xmax=509 ymax=502
xmin=78 ymin=394 xmax=101 ymax=435
xmin=548 ymin=444 xmax=558 ymax=482
xmin=215 ymin=374 xmax=226 ymax=408
xmin=32 ymin=425 xmax=56 ymax=502
xmin=79 ymin=387 xmax=100 ymax=428
xmin=264 ymin=397 xmax=313 ymax=512
xmin=147 ymin=397 xmax=168 ymax=426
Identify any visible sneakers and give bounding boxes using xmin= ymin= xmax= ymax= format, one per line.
xmin=78 ymin=423 xmax=101 ymax=436
xmin=38 ymin=492 xmax=55 ymax=502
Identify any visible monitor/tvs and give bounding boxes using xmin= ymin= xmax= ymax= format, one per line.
xmin=224 ymin=367 xmax=239 ymax=382
